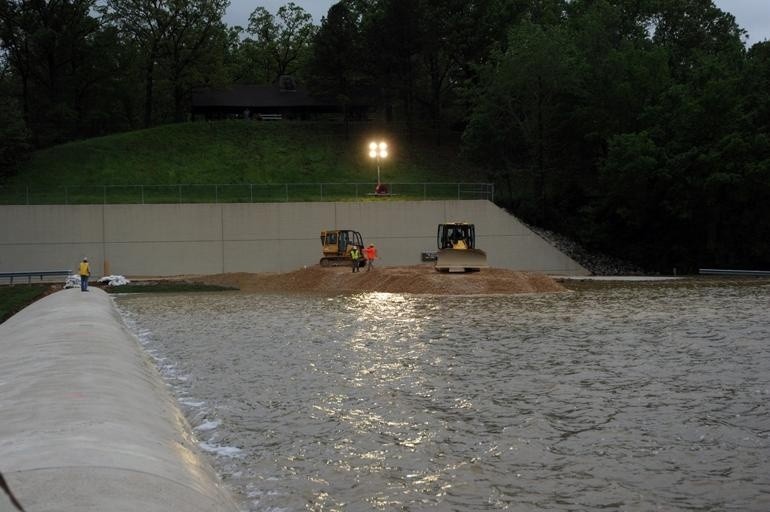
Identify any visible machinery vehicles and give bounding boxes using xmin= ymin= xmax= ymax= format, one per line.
xmin=318 ymin=228 xmax=366 ymax=268
xmin=421 ymin=221 xmax=489 ymax=274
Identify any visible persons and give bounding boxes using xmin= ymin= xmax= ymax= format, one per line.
xmin=350 ymin=246 xmax=361 ymax=273
xmin=364 ymin=244 xmax=376 ymax=272
xmin=78 ymin=257 xmax=91 ymax=291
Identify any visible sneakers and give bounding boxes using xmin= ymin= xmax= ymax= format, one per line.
xmin=352 ymin=272 xmax=356 ymax=273
xmin=81 ymin=290 xmax=84 ymax=291
xmin=357 ymin=271 xmax=360 ymax=272
xmin=85 ymin=290 xmax=88 ymax=291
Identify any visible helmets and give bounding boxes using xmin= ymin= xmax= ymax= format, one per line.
xmin=353 ymin=246 xmax=357 ymax=249
xmin=84 ymin=257 xmax=87 ymax=260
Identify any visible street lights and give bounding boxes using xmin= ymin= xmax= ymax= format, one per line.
xmin=367 ymin=140 xmax=389 ymax=184
